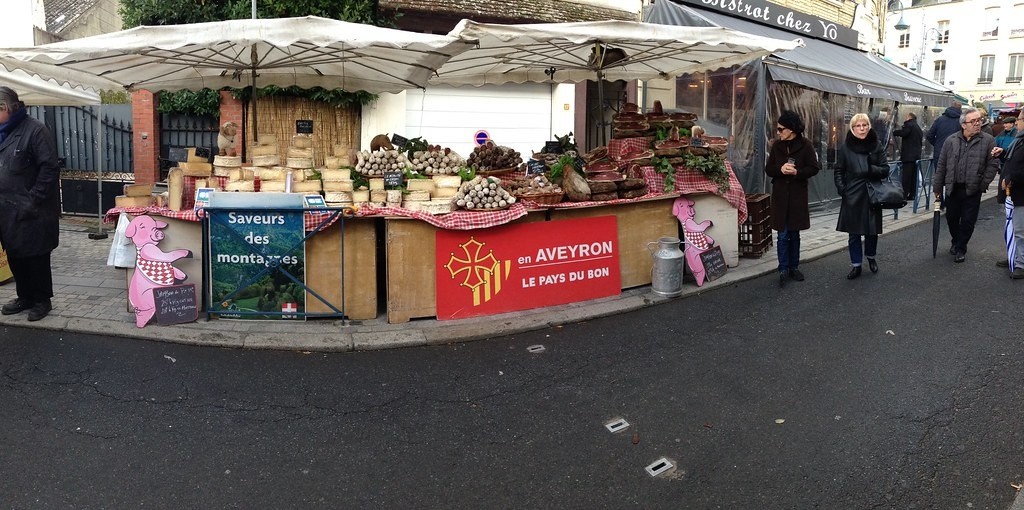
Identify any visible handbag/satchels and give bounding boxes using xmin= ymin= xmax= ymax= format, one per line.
xmin=866 ymin=175 xmax=907 ymax=209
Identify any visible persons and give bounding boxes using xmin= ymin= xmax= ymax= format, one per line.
xmin=0 ymin=86 xmax=61 ymax=321
xmin=893 ymin=112 xmax=923 ymax=201
xmin=764 ymin=110 xmax=819 ymax=283
xmin=834 ymin=113 xmax=888 ymax=278
xmin=932 ymin=107 xmax=998 ymax=262
xmin=991 ymin=107 xmax=1024 ymax=278
xmin=977 ymin=109 xmax=1019 ymax=172
xmin=927 ymin=100 xmax=963 ymax=172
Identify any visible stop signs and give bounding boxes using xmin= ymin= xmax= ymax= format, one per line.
xmin=474 ymin=130 xmax=491 ymax=147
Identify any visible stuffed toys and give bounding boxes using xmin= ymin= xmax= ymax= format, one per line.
xmin=217 ymin=121 xmax=238 ymax=156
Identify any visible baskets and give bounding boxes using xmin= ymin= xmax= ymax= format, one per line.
xmin=516 ymin=193 xmax=564 ymax=204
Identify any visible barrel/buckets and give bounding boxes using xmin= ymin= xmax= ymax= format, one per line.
xmin=647 ymin=236 xmax=692 ymax=298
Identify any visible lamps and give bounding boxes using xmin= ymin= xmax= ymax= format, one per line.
xmin=887 ymin=0 xmax=908 ymax=30
xmin=925 ymin=28 xmax=943 ymax=52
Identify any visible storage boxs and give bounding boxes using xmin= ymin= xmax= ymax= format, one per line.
xmin=739 ymin=192 xmax=773 ymax=260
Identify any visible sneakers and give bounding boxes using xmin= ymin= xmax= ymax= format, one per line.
xmin=2 ymin=298 xmax=35 ymax=315
xmin=29 ymin=298 xmax=52 ymax=320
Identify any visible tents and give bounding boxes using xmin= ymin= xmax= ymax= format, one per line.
xmin=0 ymin=64 xmax=103 ymax=233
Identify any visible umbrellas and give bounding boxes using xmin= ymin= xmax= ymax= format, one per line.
xmin=0 ymin=13 xmax=478 ymax=162
xmin=933 ymin=194 xmax=941 ymax=259
xmin=428 ymin=16 xmax=803 ymax=148
xmin=1004 ymin=186 xmax=1016 ymax=278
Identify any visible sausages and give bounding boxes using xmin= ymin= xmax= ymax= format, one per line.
xmin=355 ymin=142 xmax=523 ymax=208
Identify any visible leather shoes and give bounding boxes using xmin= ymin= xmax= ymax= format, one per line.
xmin=954 ymin=252 xmax=965 ymax=262
xmin=847 ymin=265 xmax=862 ymax=278
xmin=867 ymin=257 xmax=878 ymax=272
xmin=950 ymin=244 xmax=957 ymax=254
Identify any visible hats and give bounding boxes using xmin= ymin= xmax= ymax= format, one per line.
xmin=1002 ymin=117 xmax=1016 ymax=122
xmin=778 ymin=110 xmax=805 ymax=134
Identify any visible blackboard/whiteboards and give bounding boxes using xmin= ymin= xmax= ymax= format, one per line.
xmin=169 ymin=148 xmax=188 ymax=162
xmin=527 ymin=160 xmax=546 ymax=173
xmin=699 ymin=245 xmax=728 ymax=281
xmin=384 ymin=171 xmax=402 ymax=185
xmin=392 ymin=133 xmax=408 ymax=148
xmin=153 ymin=283 xmax=198 ymax=325
xmin=296 ymin=120 xmax=313 ymax=133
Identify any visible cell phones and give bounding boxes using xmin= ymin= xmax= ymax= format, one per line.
xmin=787 ymin=158 xmax=796 ymax=164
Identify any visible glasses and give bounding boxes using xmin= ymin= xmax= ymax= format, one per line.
xmin=964 ymin=119 xmax=980 ymax=124
xmin=778 ymin=127 xmax=788 ymax=132
xmin=852 ymin=123 xmax=869 ymax=128
xmin=1015 ymin=118 xmax=1024 ymax=122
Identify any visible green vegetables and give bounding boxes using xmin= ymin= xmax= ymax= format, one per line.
xmin=307 ymin=137 xmax=475 ymax=194
xmin=651 ymin=128 xmax=731 ymax=194
xmin=549 ymin=155 xmax=575 ymax=178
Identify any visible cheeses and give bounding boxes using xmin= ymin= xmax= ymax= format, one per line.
xmin=115 ymin=133 xmax=462 ymax=215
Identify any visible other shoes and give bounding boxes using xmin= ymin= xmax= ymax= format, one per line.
xmin=1010 ymin=268 xmax=1024 ymax=278
xmin=790 ymin=269 xmax=804 ymax=280
xmin=996 ymin=259 xmax=1008 ymax=267
xmin=780 ymin=272 xmax=787 ymax=287
